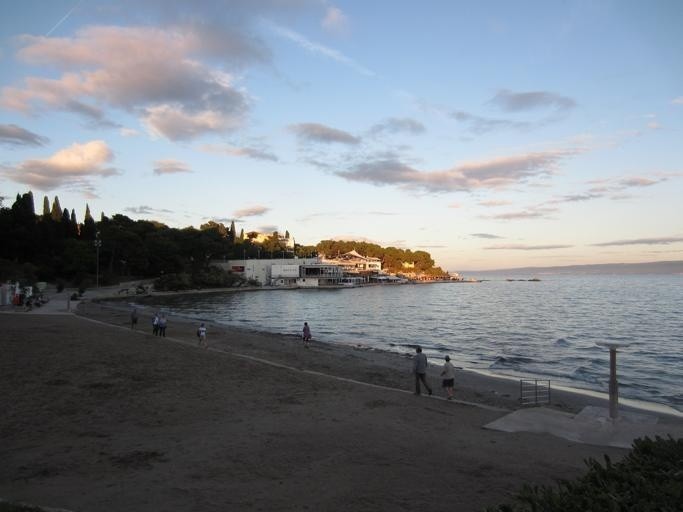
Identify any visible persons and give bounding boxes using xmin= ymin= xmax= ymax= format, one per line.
xmin=411 ymin=345 xmax=431 ymax=397
xmin=130 ymin=308 xmax=138 ymax=330
xmin=158 ymin=314 xmax=168 ymax=338
xmin=440 ymin=355 xmax=455 ymax=399
xmin=302 ymin=322 xmax=311 ymax=345
xmin=151 ymin=312 xmax=159 ymax=336
xmin=196 ymin=323 xmax=209 ymax=348
xmin=12 ymin=278 xmax=48 ymax=311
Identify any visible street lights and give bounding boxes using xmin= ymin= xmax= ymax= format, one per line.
xmin=94 ymin=232 xmax=101 ymax=289
xmin=189 ymin=256 xmax=193 ymax=289
xmin=205 ymin=255 xmax=210 ymax=271
